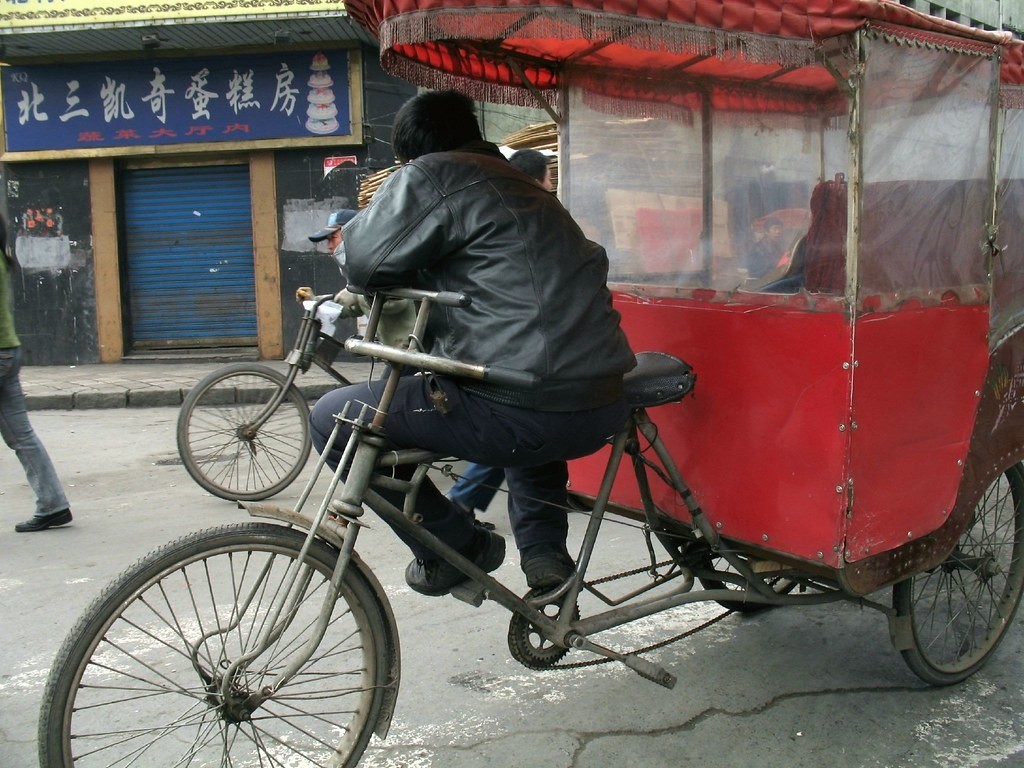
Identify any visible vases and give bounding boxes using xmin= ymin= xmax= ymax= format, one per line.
xmin=15 ymin=235 xmax=70 ymax=270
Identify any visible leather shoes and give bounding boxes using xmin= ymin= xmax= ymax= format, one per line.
xmin=15 ymin=508 xmax=72 ymax=533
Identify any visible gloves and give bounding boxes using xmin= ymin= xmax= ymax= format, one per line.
xmin=295 ymin=287 xmax=316 ymax=304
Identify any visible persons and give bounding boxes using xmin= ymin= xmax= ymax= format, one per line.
xmin=295 ymin=87 xmax=639 ymax=598
xmin=741 ymin=217 xmax=784 ymax=280
xmin=0 ymin=214 xmax=73 ymax=532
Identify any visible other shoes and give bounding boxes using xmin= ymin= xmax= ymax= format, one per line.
xmin=520 ymin=539 xmax=584 ymax=593
xmin=445 ymin=492 xmax=495 ymax=531
xmin=405 ymin=530 xmax=506 ymax=597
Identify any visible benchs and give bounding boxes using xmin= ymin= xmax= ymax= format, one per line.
xmin=760 ymin=177 xmax=1024 ymax=458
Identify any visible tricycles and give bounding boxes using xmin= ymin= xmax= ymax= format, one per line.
xmin=40 ymin=1 xmax=1024 ymax=767
xmin=173 ymin=291 xmax=362 ymax=507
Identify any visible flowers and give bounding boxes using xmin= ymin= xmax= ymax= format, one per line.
xmin=17 ymin=209 xmax=57 ymax=236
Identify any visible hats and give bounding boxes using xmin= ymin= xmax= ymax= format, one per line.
xmin=308 ymin=209 xmax=359 ymax=243
